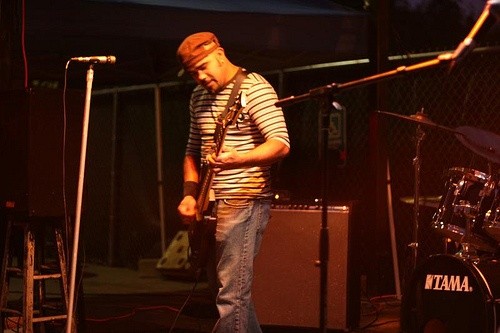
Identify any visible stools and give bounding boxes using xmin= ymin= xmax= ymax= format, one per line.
xmin=0 ymin=212 xmax=77 ymax=333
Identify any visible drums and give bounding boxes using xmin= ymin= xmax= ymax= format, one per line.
xmin=482 ymin=202 xmax=500 ymax=238
xmin=399 ymin=250 xmax=500 ymax=333
xmin=445 ymin=167 xmax=496 ymax=218
xmin=431 ymin=167 xmax=496 ymax=250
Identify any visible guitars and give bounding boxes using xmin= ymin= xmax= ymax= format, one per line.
xmin=188 ymin=90 xmax=247 ymax=267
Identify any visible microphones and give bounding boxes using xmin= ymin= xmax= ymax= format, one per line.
xmin=447 ymin=0 xmax=500 ymax=79
xmin=70 ymin=55 xmax=116 ymax=65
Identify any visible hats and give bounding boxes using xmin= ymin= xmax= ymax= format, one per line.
xmin=176 ymin=31 xmax=221 ymax=69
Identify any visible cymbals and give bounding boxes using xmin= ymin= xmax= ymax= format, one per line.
xmin=400 ymin=193 xmax=442 ymax=209
xmin=375 ymin=109 xmax=467 ymax=135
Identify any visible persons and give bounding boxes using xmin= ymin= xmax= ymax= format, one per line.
xmin=177 ymin=32 xmax=291 ymax=333
